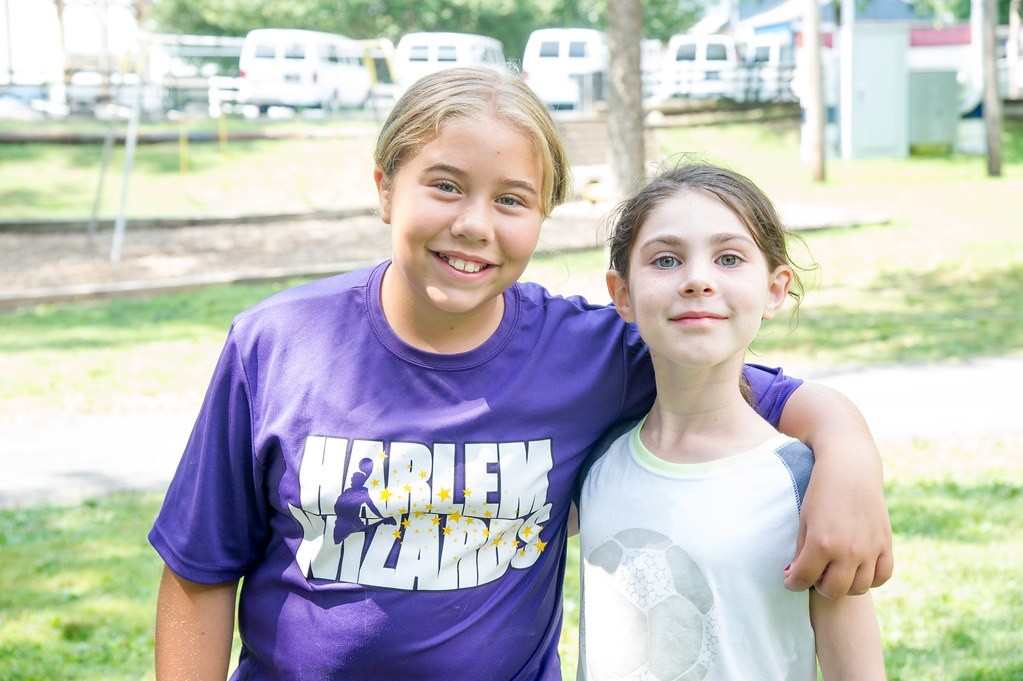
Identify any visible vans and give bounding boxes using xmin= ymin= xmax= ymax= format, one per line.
xmin=237 ymin=25 xmax=370 ymax=113
xmin=664 ymin=32 xmax=740 ymax=102
xmin=397 ymin=30 xmax=506 ymax=98
xmin=517 ymin=26 xmax=608 ymax=111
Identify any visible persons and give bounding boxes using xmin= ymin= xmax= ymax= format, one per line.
xmin=147 ymin=61 xmax=896 ymax=681
xmin=576 ymin=160 xmax=893 ymax=681
